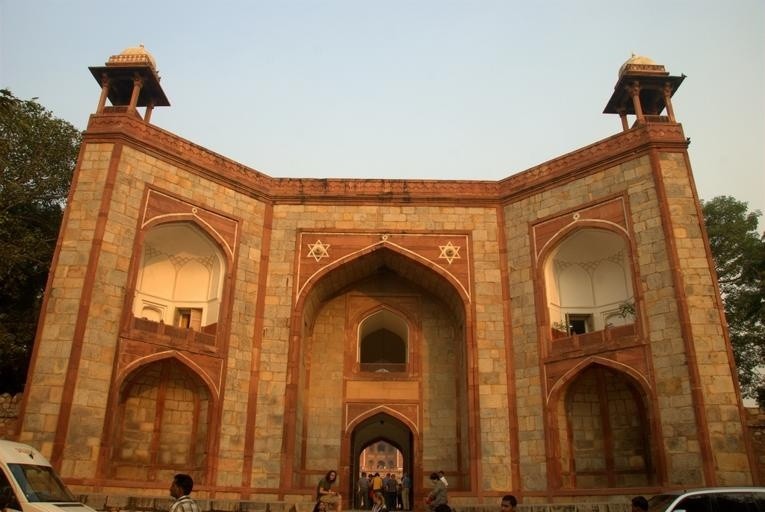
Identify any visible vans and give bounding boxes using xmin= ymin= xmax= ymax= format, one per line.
xmin=0 ymin=440 xmax=97 ymax=512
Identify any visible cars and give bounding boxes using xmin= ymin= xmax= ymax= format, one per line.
xmin=648 ymin=486 xmax=765 ymax=512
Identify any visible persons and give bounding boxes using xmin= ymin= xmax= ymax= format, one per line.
xmin=357 ymin=472 xmax=410 ymax=511
xmin=498 ymin=495 xmax=519 ymax=512
xmin=631 ymin=496 xmax=649 ymax=512
xmin=429 ymin=472 xmax=448 ymax=512
xmin=168 ymin=473 xmax=202 ymax=512
xmin=312 ymin=470 xmax=337 ymax=512
xmin=438 ymin=470 xmax=448 ymax=488
